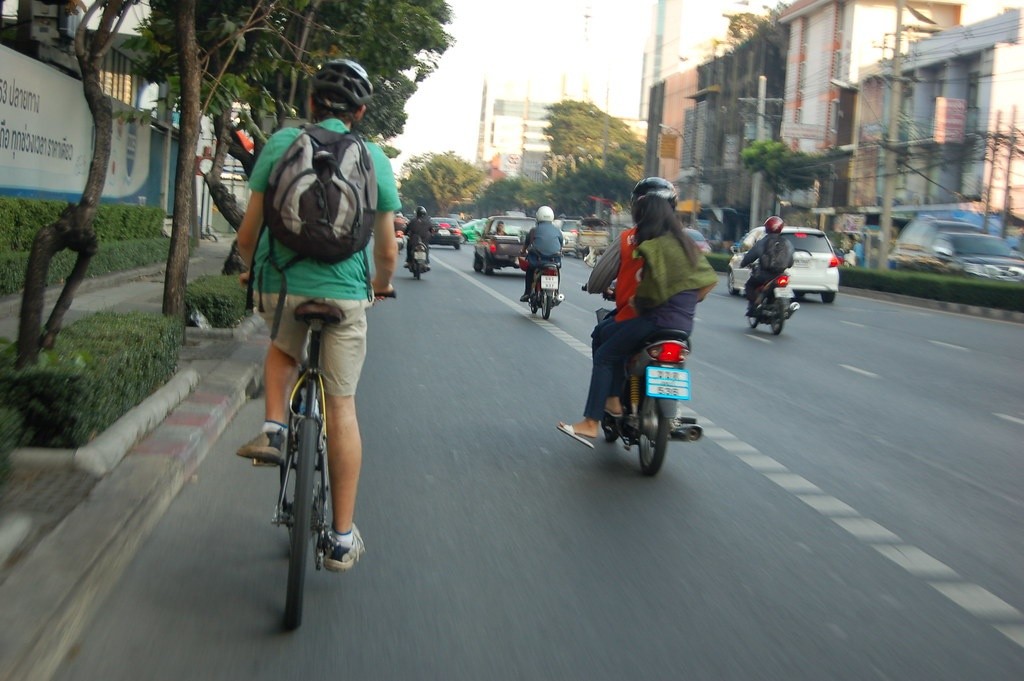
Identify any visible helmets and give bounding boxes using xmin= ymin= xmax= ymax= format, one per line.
xmin=315 ymin=57 xmax=373 ymax=106
xmin=535 ymin=207 xmax=554 ymax=223
xmin=630 ymin=178 xmax=680 ymax=224
xmin=416 ymin=206 xmax=427 ymax=215
xmin=766 ymin=216 xmax=788 ymax=234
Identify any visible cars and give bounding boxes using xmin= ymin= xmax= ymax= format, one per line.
xmin=682 ymin=220 xmax=723 ymax=257
xmin=430 ymin=217 xmax=465 ymax=250
xmin=726 ymin=225 xmax=840 ymax=305
xmin=460 ymin=217 xmax=486 ymax=243
xmin=555 ymin=217 xmax=580 ymax=254
xmin=888 ymin=215 xmax=1023 ymax=281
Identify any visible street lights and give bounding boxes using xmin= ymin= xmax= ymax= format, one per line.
xmin=587 ymin=109 xmax=609 ymax=217
xmin=829 ymin=77 xmax=910 ymax=273
xmin=658 ymin=122 xmax=700 ymax=229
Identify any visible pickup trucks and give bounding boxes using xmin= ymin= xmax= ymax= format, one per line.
xmin=473 ymin=211 xmax=537 ymax=276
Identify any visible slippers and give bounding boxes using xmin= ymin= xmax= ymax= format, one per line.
xmin=603 ymin=400 xmax=623 ymax=418
xmin=557 ymin=421 xmax=596 ymax=450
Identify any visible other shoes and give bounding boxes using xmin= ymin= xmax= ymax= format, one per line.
xmin=746 ymin=309 xmax=757 ymax=316
xmin=520 ymin=294 xmax=529 ymax=300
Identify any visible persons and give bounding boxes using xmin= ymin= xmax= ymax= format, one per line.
xmin=843 ymin=242 xmax=857 ymax=267
xmin=494 ymin=221 xmax=508 ymax=235
xmin=404 ymin=206 xmax=437 ymax=268
xmin=235 ymin=58 xmax=402 ymax=570
xmin=739 ymin=216 xmax=795 ymax=317
xmin=519 ymin=206 xmax=564 ymax=301
xmin=556 ymin=174 xmax=720 ymax=452
xmin=853 ymin=234 xmax=865 ymax=268
xmin=394 ymin=211 xmax=407 ymax=238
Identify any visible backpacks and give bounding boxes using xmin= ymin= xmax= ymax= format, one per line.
xmin=262 ymin=113 xmax=377 ymax=260
xmin=765 ymin=238 xmax=791 ymax=273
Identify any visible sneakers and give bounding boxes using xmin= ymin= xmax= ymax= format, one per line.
xmin=323 ymin=522 xmax=364 ymax=573
xmin=238 ymin=427 xmax=287 ymax=465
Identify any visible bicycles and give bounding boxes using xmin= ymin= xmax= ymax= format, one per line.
xmin=241 ymin=272 xmax=396 ymax=628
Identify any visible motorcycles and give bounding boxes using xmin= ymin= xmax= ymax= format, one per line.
xmin=517 ymin=250 xmax=564 ymax=321
xmin=403 ymin=230 xmax=431 ymax=281
xmin=577 ymin=282 xmax=705 ymax=480
xmin=574 ymin=217 xmax=611 ymax=257
xmin=742 ymin=263 xmax=801 ymax=335
